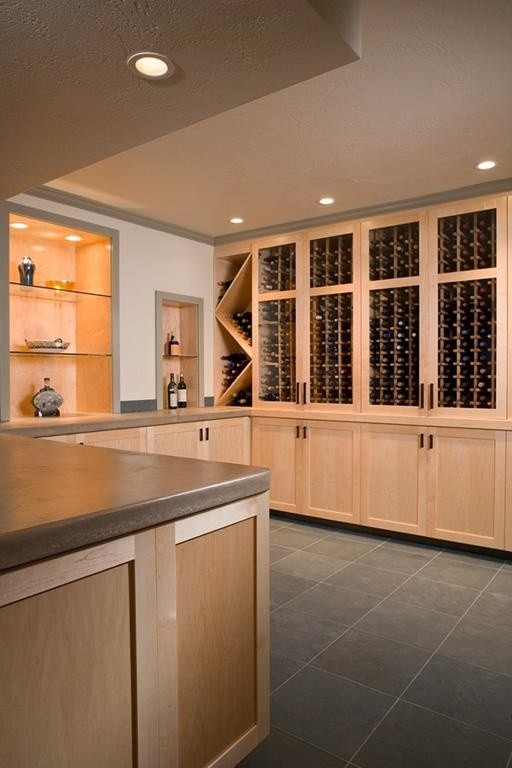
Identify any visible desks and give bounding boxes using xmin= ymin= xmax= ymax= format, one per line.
xmin=0 ymin=430 xmax=271 ymax=767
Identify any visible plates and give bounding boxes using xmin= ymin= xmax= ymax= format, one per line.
xmin=44 ymin=280 xmax=78 ymax=290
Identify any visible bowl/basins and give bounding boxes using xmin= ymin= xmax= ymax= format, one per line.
xmin=27 ymin=339 xmax=70 ymax=352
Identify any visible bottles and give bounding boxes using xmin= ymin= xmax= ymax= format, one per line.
xmin=262 ymin=212 xmax=496 ymax=409
xmin=31 ymin=376 xmax=63 ymax=416
xmin=219 ymin=354 xmax=251 ymax=406
xmin=166 ymin=373 xmax=178 ymax=409
xmin=179 ymin=374 xmax=188 ymax=408
xmin=169 ymin=333 xmax=180 ymax=355
xmin=216 ymin=279 xmax=251 ymax=348
xmin=17 ymin=257 xmax=35 ymax=285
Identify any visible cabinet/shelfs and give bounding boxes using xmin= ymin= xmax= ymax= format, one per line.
xmin=360 ymin=191 xmax=507 ymax=419
xmin=213 ymin=237 xmax=258 ymax=410
xmin=147 ymin=416 xmax=251 ymax=466
xmin=360 ymin=423 xmax=507 ymax=558
xmin=37 ymin=427 xmax=147 ymax=454
xmin=9 ymin=281 xmax=112 ymax=357
xmin=258 ymin=219 xmax=361 ymax=412
xmin=251 ymin=417 xmax=361 ymax=532
